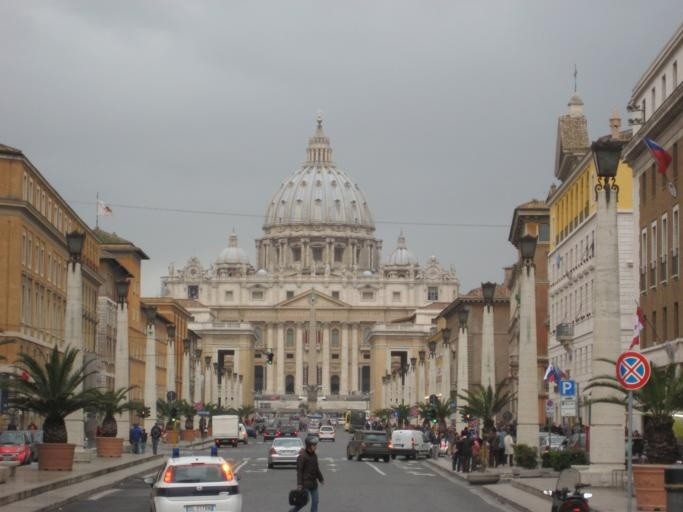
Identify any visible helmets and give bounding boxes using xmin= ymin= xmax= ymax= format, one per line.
xmin=305 ymin=436 xmax=319 ymax=449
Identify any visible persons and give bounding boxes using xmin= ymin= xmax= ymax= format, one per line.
xmin=631 ymin=430 xmax=643 ymax=458
xmin=198 ymin=415 xmax=207 ymax=440
xmin=429 ymin=424 xmax=515 ymax=474
xmin=139 ymin=428 xmax=147 ymax=453
xmin=128 ymin=423 xmax=142 ymax=455
xmin=288 ymin=434 xmax=325 ymax=511
xmin=27 ymin=421 xmax=38 ymax=431
xmin=150 ymin=422 xmax=160 ymax=455
xmin=7 ymin=420 xmax=17 ymax=431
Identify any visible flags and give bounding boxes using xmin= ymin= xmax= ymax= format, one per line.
xmin=541 ymin=363 xmax=566 ymax=386
xmin=628 ymin=297 xmax=648 ymax=350
xmin=96 ymin=199 xmax=112 ymax=218
xmin=639 ymin=134 xmax=673 ymax=175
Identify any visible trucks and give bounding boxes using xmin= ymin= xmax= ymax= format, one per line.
xmin=212 ymin=415 xmax=240 ymax=448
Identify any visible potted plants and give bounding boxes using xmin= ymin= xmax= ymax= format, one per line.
xmin=1 ymin=342 xmax=213 ymax=471
xmin=414 ymin=359 xmax=590 ymax=478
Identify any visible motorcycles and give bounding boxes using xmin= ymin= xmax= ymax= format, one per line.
xmin=543 ymin=468 xmax=592 ymax=512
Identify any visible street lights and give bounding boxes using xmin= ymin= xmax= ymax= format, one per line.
xmin=382 ymin=279 xmax=497 ymax=434
xmin=512 ymin=233 xmax=545 ymax=468
xmin=586 ymin=134 xmax=627 ymax=465
xmin=64 ymin=228 xmax=243 ymax=443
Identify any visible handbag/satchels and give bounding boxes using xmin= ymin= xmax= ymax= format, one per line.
xmin=288 ymin=488 xmax=309 ymax=506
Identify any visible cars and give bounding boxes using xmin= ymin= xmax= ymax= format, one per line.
xmin=540 ymin=431 xmax=586 ymax=458
xmin=268 ymin=437 xmax=305 ymax=469
xmin=143 ymin=455 xmax=243 ymax=512
xmin=347 ymin=430 xmax=391 ymax=463
xmin=391 ymin=430 xmax=449 ymax=460
xmin=239 ymin=417 xmax=336 ymax=445
xmin=329 ymin=408 xmax=367 ymax=434
xmin=0 ymin=430 xmax=43 ymax=466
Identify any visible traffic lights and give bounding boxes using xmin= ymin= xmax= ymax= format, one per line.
xmin=268 ymin=353 xmax=272 ymax=365
